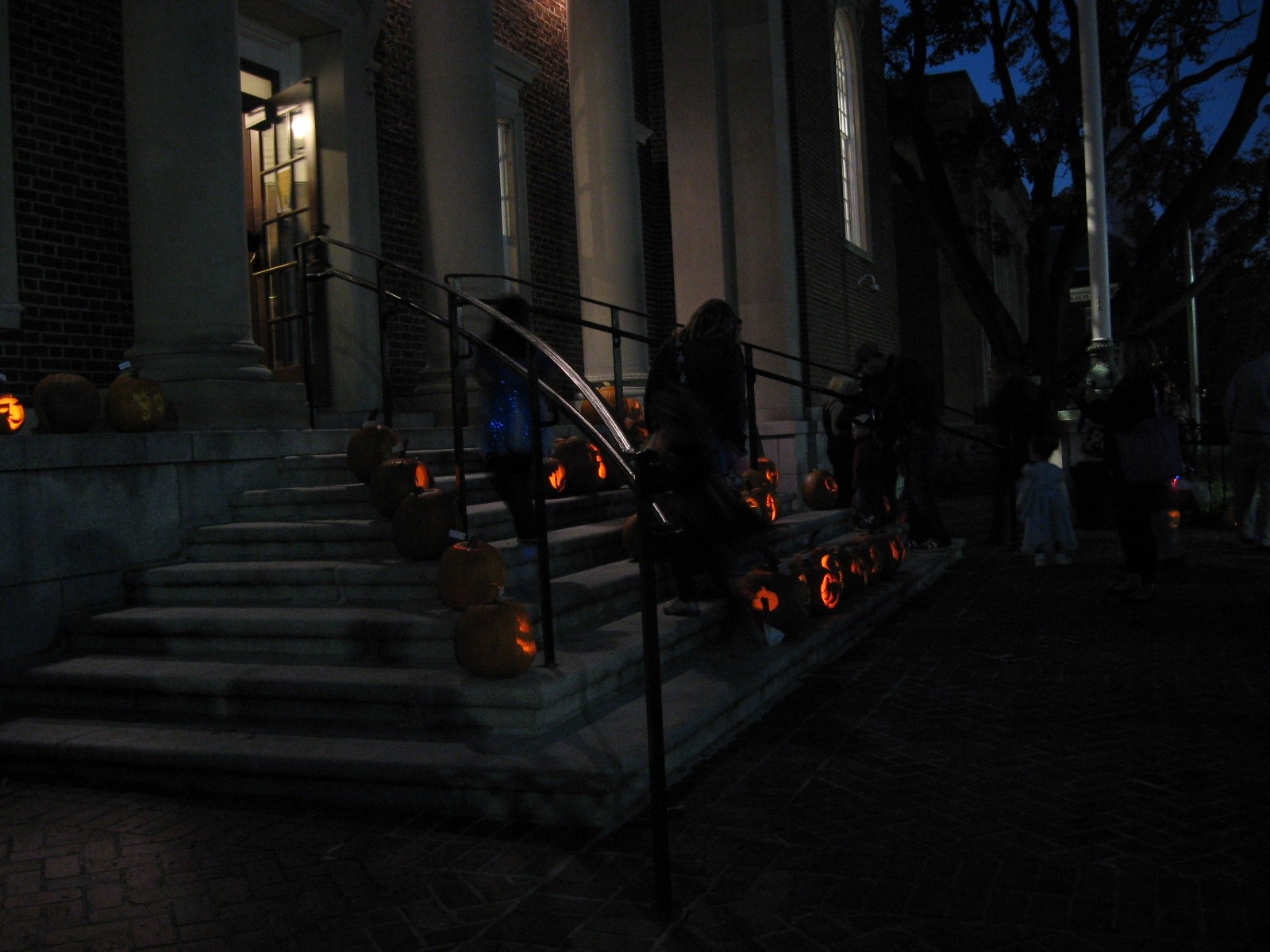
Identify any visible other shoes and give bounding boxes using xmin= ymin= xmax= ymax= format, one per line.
xmin=664 ymin=598 xmax=700 ymax=617
xmin=1115 ymin=579 xmax=1153 ymax=599
xmin=1034 ymin=554 xmax=1047 ymax=566
xmin=858 ymin=518 xmax=886 ymax=530
xmin=1054 ymin=552 xmax=1072 ymax=565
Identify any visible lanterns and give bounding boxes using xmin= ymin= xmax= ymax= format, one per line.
xmin=0 ymin=392 xmax=26 ymax=434
xmin=540 ymin=382 xmax=651 ymax=497
xmin=737 ymin=457 xmax=907 ymax=641
xmin=104 ymin=378 xmax=164 ymax=433
xmin=1158 ymin=508 xmax=1179 ymax=534
xmin=345 ymin=424 xmax=536 ymax=676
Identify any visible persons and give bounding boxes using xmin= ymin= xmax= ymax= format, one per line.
xmin=825 ymin=343 xmax=952 ymax=553
xmin=474 ymin=293 xmax=548 ymax=542
xmin=1016 ymin=434 xmax=1079 ymax=565
xmin=637 ymin=299 xmax=759 ymax=612
xmin=1071 ymin=335 xmax=1270 ymax=599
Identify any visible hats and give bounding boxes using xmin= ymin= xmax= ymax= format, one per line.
xmin=850 ymin=413 xmax=875 ymax=432
